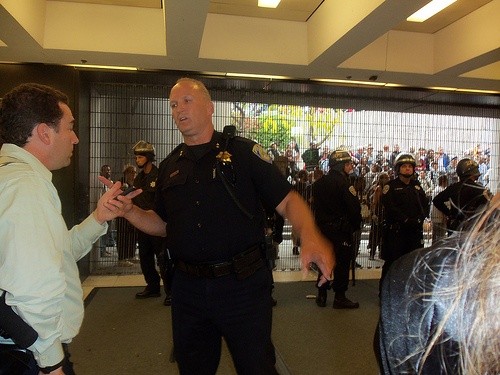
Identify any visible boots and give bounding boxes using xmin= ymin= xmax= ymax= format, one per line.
xmin=332 ymin=291 xmax=359 ymax=309
xmin=315 ymin=289 xmax=327 ymax=306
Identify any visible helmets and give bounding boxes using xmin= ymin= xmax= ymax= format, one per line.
xmin=131 ymin=140 xmax=155 ymax=163
xmin=457 ymin=158 xmax=479 ymax=177
xmin=394 ymin=153 xmax=416 ymax=172
xmin=329 ymin=149 xmax=352 ymax=171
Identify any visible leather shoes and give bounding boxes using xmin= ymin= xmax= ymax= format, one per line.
xmin=136 ymin=286 xmax=161 ymax=298
xmin=164 ymin=296 xmax=171 ymax=306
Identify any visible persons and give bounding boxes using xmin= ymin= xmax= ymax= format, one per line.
xmin=100 ymin=164 xmax=141 ymax=267
xmin=132 ymin=139 xmax=172 ymax=306
xmin=223 ymin=125 xmax=500 ymax=309
xmin=372 ymin=188 xmax=500 ymax=375
xmin=0 ymin=81 xmax=142 ymax=375
xmin=97 ymin=76 xmax=336 ymax=375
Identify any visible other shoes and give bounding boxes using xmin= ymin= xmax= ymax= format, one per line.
xmin=119 ymin=259 xmax=133 ymax=266
xmin=100 ymin=250 xmax=112 ymax=257
xmin=128 ymin=257 xmax=140 ymax=263
